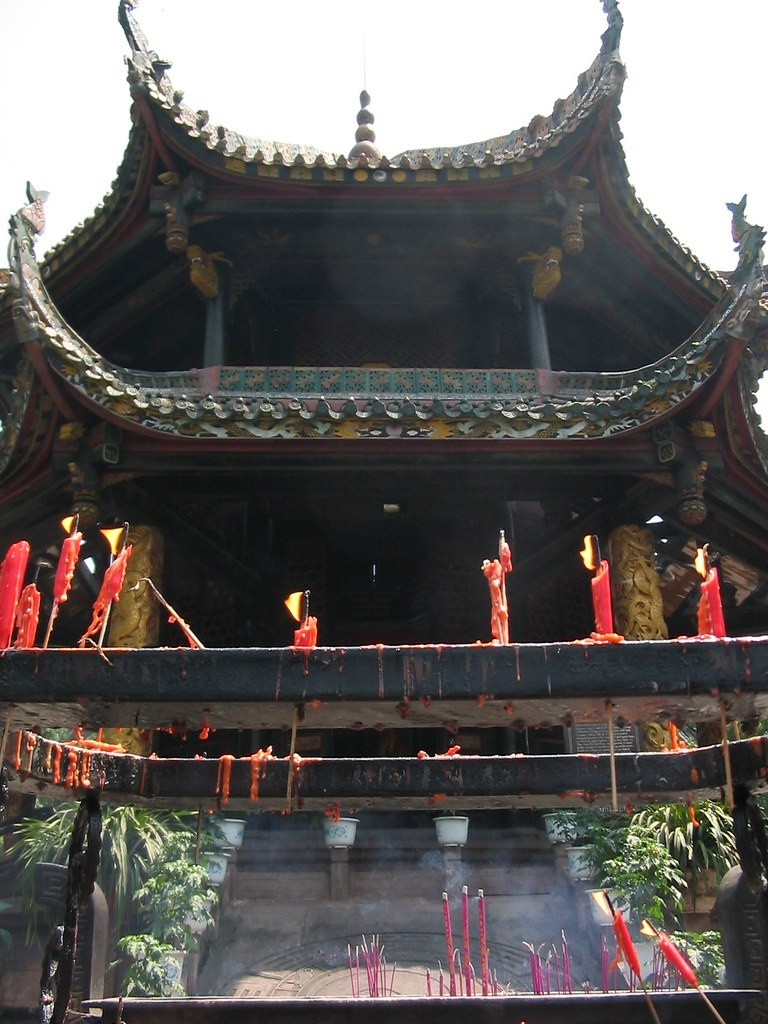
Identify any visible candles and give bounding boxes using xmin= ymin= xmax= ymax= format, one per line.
xmin=285 ymin=590 xmax=319 ymax=647
xmin=694 ymin=541 xmax=727 ymax=638
xmin=579 ymin=534 xmax=624 ymax=642
xmin=639 ymin=917 xmax=698 ymax=989
xmin=590 ymin=889 xmax=641 ymax=977
xmin=482 ymin=542 xmax=514 ymax=644
xmin=3 ymin=514 xmax=132 ymax=651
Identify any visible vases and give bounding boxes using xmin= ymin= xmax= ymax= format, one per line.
xmin=433 ymin=816 xmax=469 ymax=848
xmin=321 ymin=818 xmax=361 ymax=848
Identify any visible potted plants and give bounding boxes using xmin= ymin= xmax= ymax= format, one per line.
xmin=1 ymin=801 xmax=246 ymax=998
xmin=542 ymin=799 xmax=739 ymax=924
